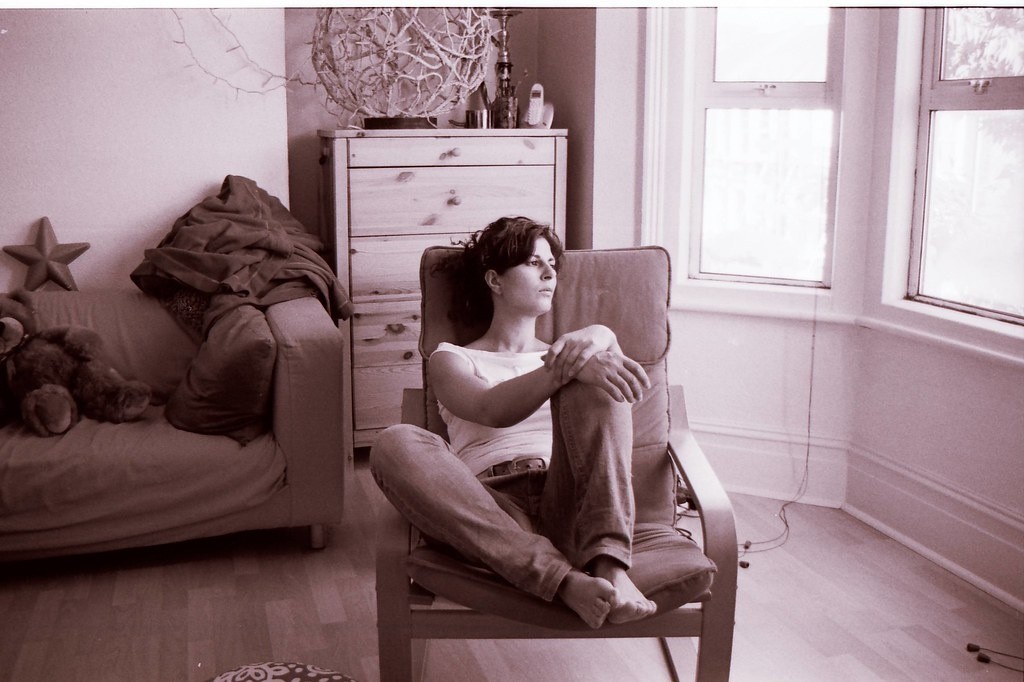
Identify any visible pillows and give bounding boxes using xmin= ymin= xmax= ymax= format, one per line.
xmin=161 ymin=298 xmax=278 ymax=449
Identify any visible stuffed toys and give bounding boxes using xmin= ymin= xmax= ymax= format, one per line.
xmin=0 ymin=288 xmax=153 ymax=438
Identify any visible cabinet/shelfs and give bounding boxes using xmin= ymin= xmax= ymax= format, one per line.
xmin=313 ymin=117 xmax=575 ymax=467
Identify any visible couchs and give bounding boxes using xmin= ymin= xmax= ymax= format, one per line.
xmin=1 ymin=284 xmax=346 ymax=578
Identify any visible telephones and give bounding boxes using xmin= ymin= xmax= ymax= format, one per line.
xmin=528 ymin=83 xmax=544 ymax=126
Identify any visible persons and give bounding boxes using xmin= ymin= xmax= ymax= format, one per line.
xmin=374 ymin=215 xmax=657 ymax=632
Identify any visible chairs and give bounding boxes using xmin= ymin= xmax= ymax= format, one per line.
xmin=373 ymin=243 xmax=743 ymax=679
